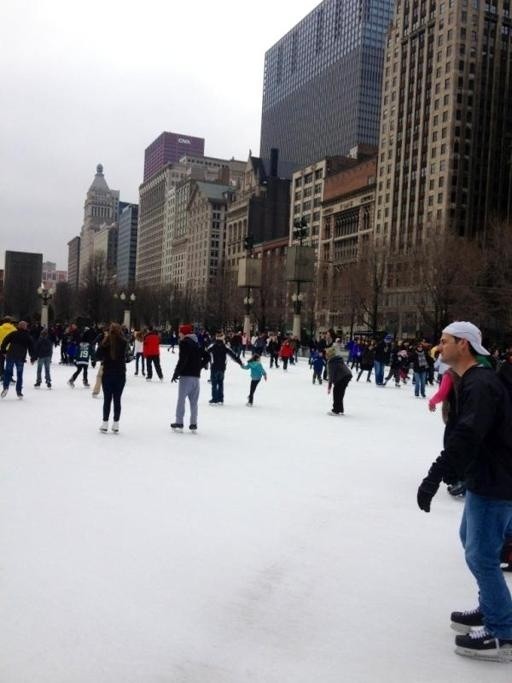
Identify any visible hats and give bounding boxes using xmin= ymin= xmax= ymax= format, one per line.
xmin=443 ymin=321 xmax=490 ymax=356
xmin=181 ymin=325 xmax=191 ymax=334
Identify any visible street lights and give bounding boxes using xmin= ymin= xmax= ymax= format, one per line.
xmin=34 ymin=281 xmax=56 ymax=334
xmin=289 ymin=216 xmax=309 ymax=360
xmin=119 ymin=290 xmax=136 ymax=330
xmin=241 ymin=232 xmax=257 ymax=347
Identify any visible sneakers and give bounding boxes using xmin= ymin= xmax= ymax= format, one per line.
xmin=189 ymin=424 xmax=197 ymax=429
xmin=34 ymin=382 xmax=51 ymax=387
xmin=455 ymin=627 xmax=512 ymax=649
xmin=171 ymin=424 xmax=183 ymax=428
xmin=208 ymin=397 xmax=224 ymax=403
xmin=1 ymin=388 xmax=23 ymax=397
xmin=451 ymin=607 xmax=485 ymax=625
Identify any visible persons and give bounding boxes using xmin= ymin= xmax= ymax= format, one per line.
xmin=1 ymin=317 xmax=450 ymax=435
xmin=428 ymin=367 xmax=466 ymax=499
xmin=476 ymin=341 xmax=511 ymax=391
xmin=417 ymin=321 xmax=512 ymax=663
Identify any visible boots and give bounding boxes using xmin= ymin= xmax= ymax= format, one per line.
xmin=112 ymin=422 xmax=119 ymax=430
xmin=99 ymin=420 xmax=108 ymax=430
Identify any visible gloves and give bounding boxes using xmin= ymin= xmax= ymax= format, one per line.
xmin=417 ymin=469 xmax=443 ymax=512
